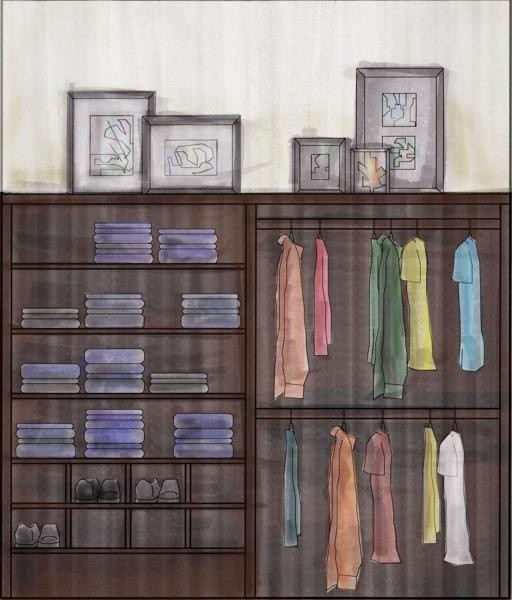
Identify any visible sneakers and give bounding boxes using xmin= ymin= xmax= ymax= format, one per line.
xmin=97 ymin=478 xmax=121 ymax=503
xmin=134 ymin=476 xmax=162 ymax=503
xmin=73 ymin=477 xmax=100 ymax=503
xmin=37 ymin=524 xmax=60 ymax=548
xmin=157 ymin=479 xmax=181 ymax=503
xmin=15 ymin=521 xmax=40 ymax=548
